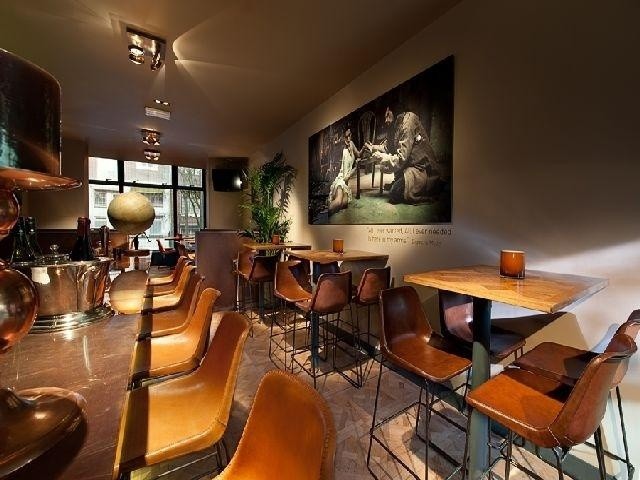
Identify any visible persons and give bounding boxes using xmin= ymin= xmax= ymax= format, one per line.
xmin=367 ymin=102 xmax=438 ymax=204
xmin=329 ymin=127 xmax=373 ymax=214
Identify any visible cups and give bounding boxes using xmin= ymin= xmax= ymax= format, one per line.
xmin=272 ymin=235 xmax=280 ymax=244
xmin=500 ymin=249 xmax=526 ymax=280
xmin=332 ymin=238 xmax=343 ymax=254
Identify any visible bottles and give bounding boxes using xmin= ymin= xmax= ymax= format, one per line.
xmin=10 ymin=215 xmax=39 ymax=263
xmin=69 ymin=215 xmax=97 ymax=261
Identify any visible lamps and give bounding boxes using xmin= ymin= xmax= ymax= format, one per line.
xmin=142 ymin=146 xmax=162 ymax=163
xmin=139 ymin=127 xmax=162 ymax=146
xmin=143 ymin=96 xmax=172 ymax=122
xmin=123 ymin=24 xmax=167 ymax=72
